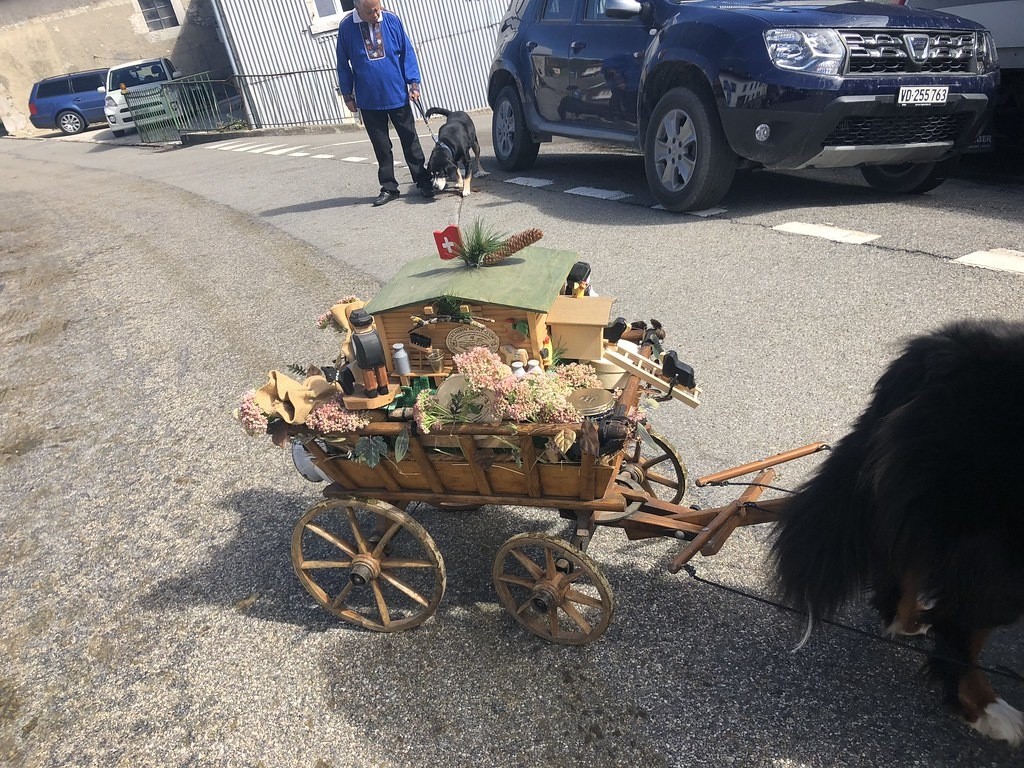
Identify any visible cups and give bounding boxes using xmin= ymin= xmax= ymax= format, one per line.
xmin=425 ymin=349 xmax=445 ymax=373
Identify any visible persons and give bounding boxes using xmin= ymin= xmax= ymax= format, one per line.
xmin=336 ymin=0 xmax=434 ymax=205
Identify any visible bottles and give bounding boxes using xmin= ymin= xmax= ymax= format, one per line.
xmin=511 ymin=361 xmax=526 ymax=376
xmin=393 ymin=342 xmax=411 ymax=375
xmin=527 ymin=359 xmax=542 ymax=374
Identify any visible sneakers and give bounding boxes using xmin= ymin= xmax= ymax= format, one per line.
xmin=373 ymin=186 xmax=400 ymax=206
xmin=420 ymin=184 xmax=436 ymax=197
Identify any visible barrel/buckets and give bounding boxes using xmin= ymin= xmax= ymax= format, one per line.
xmin=292 ymin=440 xmax=329 ymax=483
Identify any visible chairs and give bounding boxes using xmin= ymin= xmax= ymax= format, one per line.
xmin=120 ymin=73 xmax=137 ymax=86
xmin=151 ymin=65 xmax=162 ymax=81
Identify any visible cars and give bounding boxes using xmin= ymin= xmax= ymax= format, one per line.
xmin=97 ymin=57 xmax=198 ymax=138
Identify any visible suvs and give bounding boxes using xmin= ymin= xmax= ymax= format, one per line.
xmin=28 ymin=67 xmax=147 ymax=136
xmin=486 ymin=0 xmax=1003 ymax=214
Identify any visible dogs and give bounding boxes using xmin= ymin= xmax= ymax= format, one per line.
xmin=763 ymin=317 xmax=1024 ymax=748
xmin=424 ymin=107 xmax=487 ymax=197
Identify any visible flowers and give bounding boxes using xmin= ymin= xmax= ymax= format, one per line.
xmin=233 ymin=295 xmax=658 ymax=464
